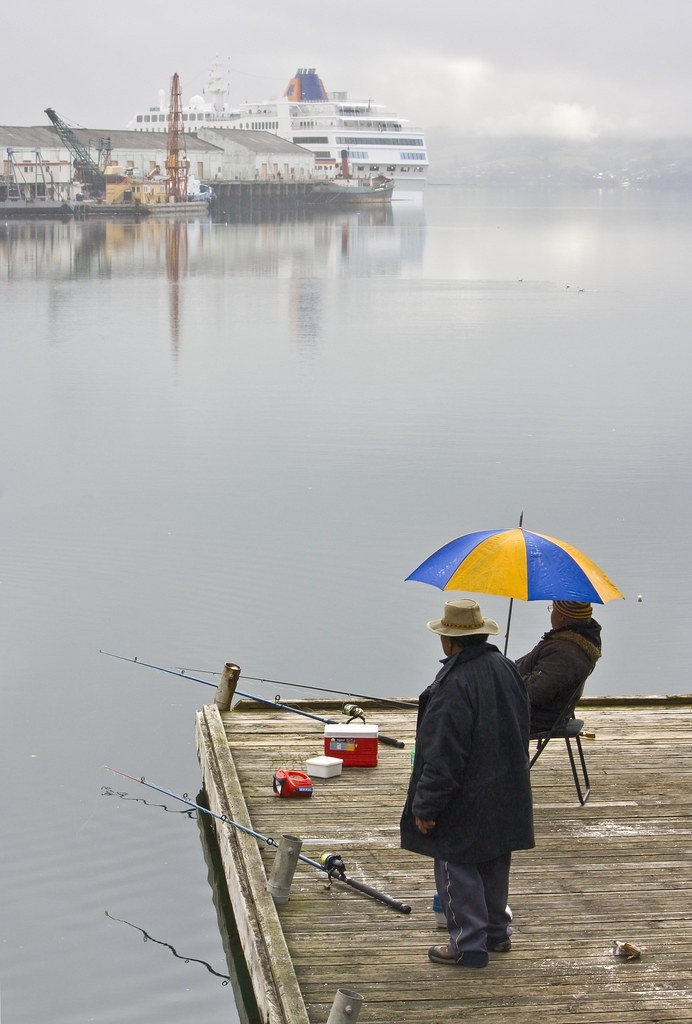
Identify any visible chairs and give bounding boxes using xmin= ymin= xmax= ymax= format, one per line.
xmin=528 ymin=664 xmax=595 ymax=805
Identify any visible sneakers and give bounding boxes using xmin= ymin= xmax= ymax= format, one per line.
xmin=428 ymin=945 xmax=487 ymax=968
xmin=489 ymin=938 xmax=512 ymax=951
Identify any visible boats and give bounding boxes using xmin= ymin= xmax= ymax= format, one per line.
xmin=0 ymin=163 xmax=213 ymax=215
xmin=311 ymin=176 xmax=396 ymax=201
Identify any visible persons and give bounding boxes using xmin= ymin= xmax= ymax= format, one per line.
xmin=399 ymin=598 xmax=536 ymax=968
xmin=514 ymin=600 xmax=603 ymax=735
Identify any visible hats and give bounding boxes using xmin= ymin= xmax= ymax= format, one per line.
xmin=427 ymin=598 xmax=500 ymax=637
xmin=552 ymin=600 xmax=593 ymax=619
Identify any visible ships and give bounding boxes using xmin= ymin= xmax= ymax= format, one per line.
xmin=124 ymin=67 xmax=430 ymax=199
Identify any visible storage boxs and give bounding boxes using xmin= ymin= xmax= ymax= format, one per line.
xmin=305 ymin=756 xmax=343 ymax=778
xmin=324 ymin=724 xmax=379 ymax=767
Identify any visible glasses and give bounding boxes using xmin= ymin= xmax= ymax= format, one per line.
xmin=547 ymin=605 xmax=554 ymax=613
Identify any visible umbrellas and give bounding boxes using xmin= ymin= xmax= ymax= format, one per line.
xmin=403 ymin=511 xmax=627 ymax=658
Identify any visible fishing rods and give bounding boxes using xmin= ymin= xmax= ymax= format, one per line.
xmin=107 ymin=764 xmax=411 ymax=914
xmin=174 ymin=663 xmax=596 ymax=742
xmin=97 ymin=649 xmax=406 ymax=751
